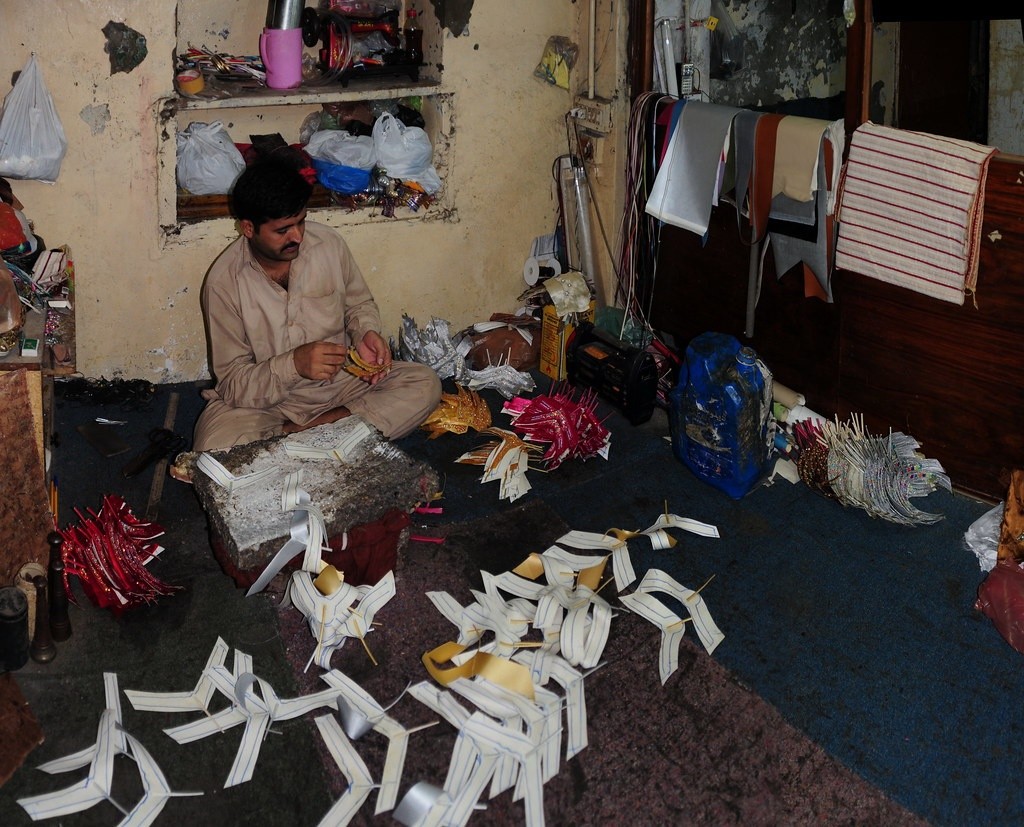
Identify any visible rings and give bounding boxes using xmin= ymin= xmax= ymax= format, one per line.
xmin=383 ymin=366 xmax=391 ymax=374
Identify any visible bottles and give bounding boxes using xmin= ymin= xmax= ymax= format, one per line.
xmin=403 ymin=8 xmax=424 ymax=65
xmin=676 ymin=330 xmax=775 ymax=500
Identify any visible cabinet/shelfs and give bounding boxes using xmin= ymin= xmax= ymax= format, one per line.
xmin=154 ymin=1 xmax=462 ymax=245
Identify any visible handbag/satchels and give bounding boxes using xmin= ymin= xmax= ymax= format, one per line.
xmin=175 ymin=120 xmax=247 ymax=196
xmin=301 ymin=130 xmax=379 ymax=173
xmin=370 ymin=112 xmax=434 ymax=179
xmin=0 ymin=52 xmax=68 ymax=187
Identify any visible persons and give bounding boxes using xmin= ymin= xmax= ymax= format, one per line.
xmin=169 ymin=154 xmax=443 ymax=483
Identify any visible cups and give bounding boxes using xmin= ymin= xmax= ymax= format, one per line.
xmin=260 ymin=27 xmax=302 ymax=89
